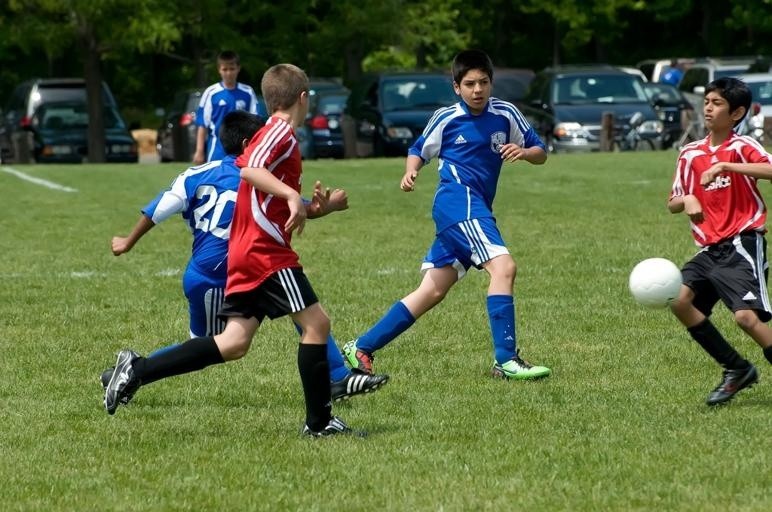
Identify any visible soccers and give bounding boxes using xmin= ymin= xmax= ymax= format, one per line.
xmin=628 ymin=257 xmax=683 ymax=312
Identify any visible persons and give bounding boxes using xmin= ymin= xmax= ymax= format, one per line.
xmin=342 ymin=48 xmax=551 ymax=380
xmin=101 ymin=109 xmax=389 ymax=406
xmin=103 ymin=62 xmax=367 ymax=442
xmin=191 ymin=49 xmax=261 ymax=165
xmin=665 ymin=77 xmax=772 ymax=405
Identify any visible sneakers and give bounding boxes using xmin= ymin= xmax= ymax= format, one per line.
xmin=105 ymin=349 xmax=143 ymax=416
xmin=705 ymin=358 xmax=760 ymax=407
xmin=98 ymin=367 xmax=114 ymax=407
xmin=342 ymin=338 xmax=377 ymax=377
xmin=301 ymin=414 xmax=367 ymax=445
xmin=329 ymin=367 xmax=390 ymax=405
xmin=489 ymin=347 xmax=549 ymax=384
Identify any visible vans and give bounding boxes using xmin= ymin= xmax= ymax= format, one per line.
xmin=677 ymin=64 xmax=749 ymax=139
xmin=640 ymin=58 xmax=693 ymax=87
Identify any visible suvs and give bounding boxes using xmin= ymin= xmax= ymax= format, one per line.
xmin=526 ymin=63 xmax=662 ymax=155
xmin=621 ymin=66 xmax=693 ymax=147
xmin=25 ymin=77 xmax=138 ymax=164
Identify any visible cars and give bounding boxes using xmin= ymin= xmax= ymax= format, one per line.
xmin=733 ymin=72 xmax=772 ymax=139
xmin=357 ymin=71 xmax=459 ymax=159
xmin=156 ymin=89 xmax=210 ymax=164
xmin=296 ymin=82 xmax=357 ymax=160
